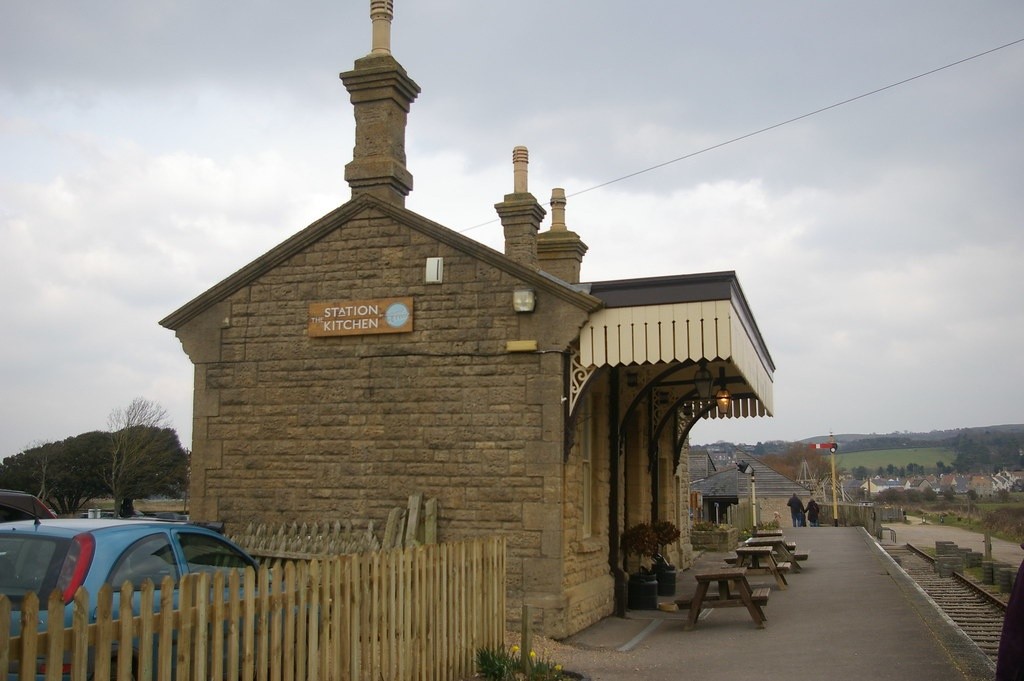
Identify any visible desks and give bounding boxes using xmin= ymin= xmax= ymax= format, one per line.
xmin=684 ymin=567 xmax=767 ymax=630
xmin=743 ymin=536 xmax=802 ymax=573
xmin=757 ymin=529 xmax=790 ymax=552
xmin=728 ymin=546 xmax=788 ymax=591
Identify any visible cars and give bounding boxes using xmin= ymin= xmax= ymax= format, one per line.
xmin=0 ymin=489 xmax=322 ymax=681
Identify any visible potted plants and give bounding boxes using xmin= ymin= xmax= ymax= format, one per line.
xmin=649 ymin=520 xmax=681 ymax=596
xmin=622 ymin=522 xmax=659 ymax=609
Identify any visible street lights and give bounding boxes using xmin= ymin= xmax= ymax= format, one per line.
xmin=736 ymin=459 xmax=757 ymax=537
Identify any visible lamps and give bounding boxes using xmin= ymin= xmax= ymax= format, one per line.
xmin=513 ymin=288 xmax=537 ymax=313
xmin=693 ymin=362 xmax=714 ymax=401
xmin=716 ymin=382 xmax=732 ymax=414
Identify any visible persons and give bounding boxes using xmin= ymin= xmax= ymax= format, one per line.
xmin=787 ymin=493 xmax=819 ymax=527
xmin=773 ymin=512 xmax=781 ymax=525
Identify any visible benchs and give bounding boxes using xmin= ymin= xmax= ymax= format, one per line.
xmin=723 ymin=557 xmax=738 ymax=564
xmin=674 ymin=594 xmax=694 ymax=610
xmin=794 ymin=550 xmax=809 ymax=560
xmin=750 ymin=587 xmax=770 ymax=607
xmin=787 ymin=542 xmax=796 ymax=550
xmin=777 ymin=562 xmax=791 ymax=573
